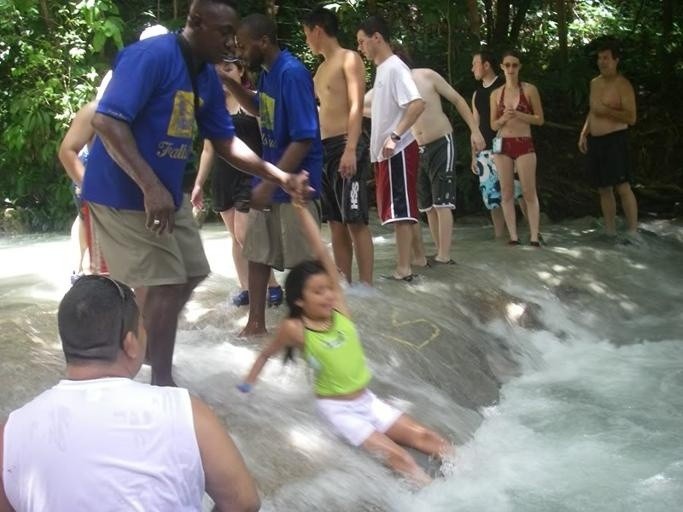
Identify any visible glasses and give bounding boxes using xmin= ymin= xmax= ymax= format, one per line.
xmin=72 ymin=274 xmax=136 ymax=350
xmin=502 ymin=62 xmax=518 ymax=68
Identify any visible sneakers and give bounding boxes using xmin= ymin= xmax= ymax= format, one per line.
xmin=379 ymin=271 xmax=415 ymax=284
xmin=269 ymin=286 xmax=283 ymax=307
xmin=234 ymin=290 xmax=250 ymax=307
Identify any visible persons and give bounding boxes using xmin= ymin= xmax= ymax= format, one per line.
xmin=233 ymin=170 xmax=459 ymax=494
xmin=578 ymin=43 xmax=640 ymax=239
xmin=1 ymin=271 xmax=261 ymax=512
